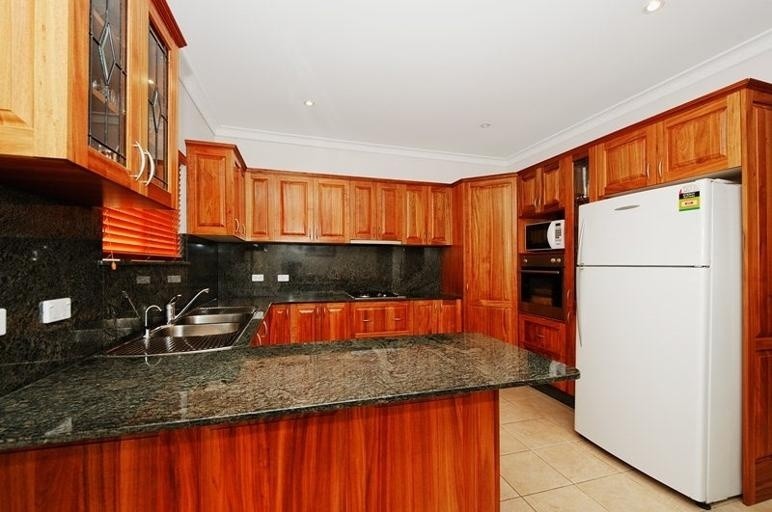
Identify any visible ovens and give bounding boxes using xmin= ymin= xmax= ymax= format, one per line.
xmin=517 ymin=250 xmax=567 ymax=324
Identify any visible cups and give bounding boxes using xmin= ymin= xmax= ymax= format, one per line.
xmin=98 ymin=79 xmax=115 ymax=105
xmin=96 ymin=142 xmax=119 ymax=163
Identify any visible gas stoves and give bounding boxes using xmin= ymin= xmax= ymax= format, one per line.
xmin=344 ymin=287 xmax=405 ymax=300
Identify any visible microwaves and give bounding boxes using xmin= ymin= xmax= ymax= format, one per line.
xmin=525 ymin=219 xmax=565 ymax=252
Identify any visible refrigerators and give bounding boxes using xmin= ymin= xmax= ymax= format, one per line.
xmin=574 ymin=176 xmax=745 ymax=509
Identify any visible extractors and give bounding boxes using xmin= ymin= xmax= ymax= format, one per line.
xmin=350 ymin=239 xmax=402 ymax=246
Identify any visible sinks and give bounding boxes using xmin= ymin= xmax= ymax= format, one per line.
xmin=175 ymin=313 xmax=252 ymax=324
xmin=150 ymin=323 xmax=243 ymax=336
xmin=76 ymin=319 xmax=138 ymax=330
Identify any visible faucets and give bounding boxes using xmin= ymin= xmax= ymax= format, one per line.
xmin=122 ymin=290 xmax=142 ymax=320
xmin=143 ymin=340 xmax=165 ymax=368
xmin=165 ymin=288 xmax=211 ymax=325
xmin=143 ymin=304 xmax=163 ymax=338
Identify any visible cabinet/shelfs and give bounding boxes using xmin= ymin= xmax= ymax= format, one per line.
xmin=276 ymin=171 xmax=350 ymax=244
xmin=518 ymin=307 xmax=571 ymax=398
xmin=402 ymin=180 xmax=454 ymax=246
xmin=351 ymin=176 xmax=401 ymax=244
xmin=187 ymin=139 xmax=247 ymax=244
xmin=245 ymin=166 xmax=276 ymax=242
xmin=517 ymin=158 xmax=565 ymax=218
xmin=442 ymin=172 xmax=518 ymax=344
xmin=0 ymin=0 xmax=188 ymax=210
xmin=592 ymin=78 xmax=772 ymax=201
xmin=249 ymin=301 xmax=461 ymax=349
